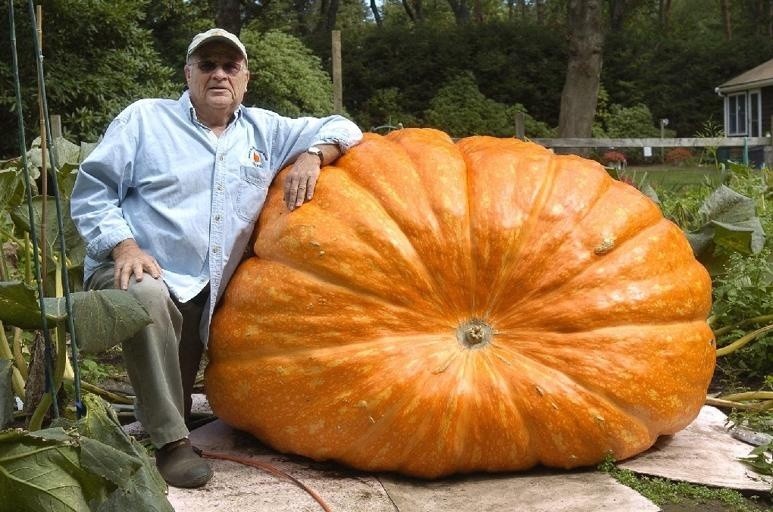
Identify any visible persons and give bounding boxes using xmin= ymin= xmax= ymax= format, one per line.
xmin=67 ymin=24 xmax=365 ymax=491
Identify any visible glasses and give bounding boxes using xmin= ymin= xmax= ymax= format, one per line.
xmin=188 ymin=60 xmax=246 ymax=75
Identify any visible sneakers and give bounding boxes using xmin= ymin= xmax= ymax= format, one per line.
xmin=155 ymin=437 xmax=214 ymax=488
xmin=184 ymin=408 xmax=218 ymax=432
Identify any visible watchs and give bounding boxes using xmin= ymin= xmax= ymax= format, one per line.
xmin=302 ymin=146 xmax=323 ymax=168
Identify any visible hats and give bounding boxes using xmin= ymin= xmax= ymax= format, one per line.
xmin=185 ymin=28 xmax=249 ymax=69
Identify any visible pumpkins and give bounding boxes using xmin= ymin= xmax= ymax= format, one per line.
xmin=202 ymin=127 xmax=717 ymax=482
